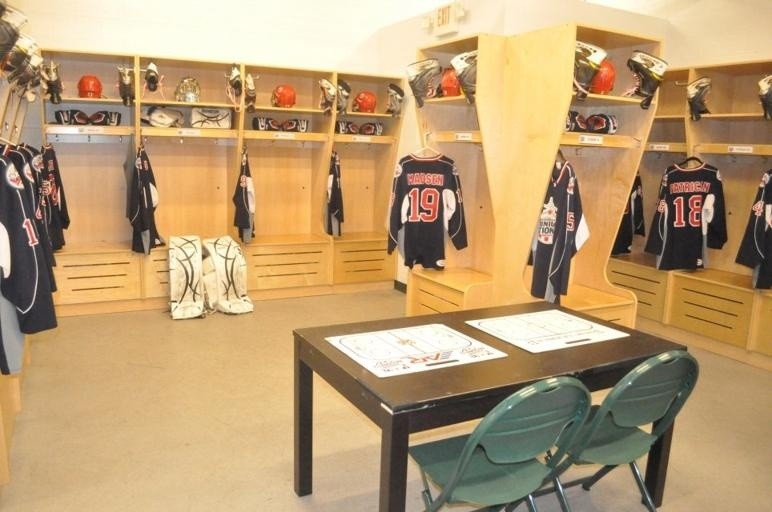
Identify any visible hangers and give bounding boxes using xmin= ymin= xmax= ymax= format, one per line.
xmin=0 ymin=137 xmax=15 ymax=147
xmin=399 ymin=129 xmax=454 ymax=167
xmin=138 ymin=136 xmax=144 ymax=157
xmin=661 ymin=142 xmax=720 ymax=179
xmin=554 ymin=149 xmax=574 ymax=182
xmin=242 ymin=139 xmax=248 ymax=154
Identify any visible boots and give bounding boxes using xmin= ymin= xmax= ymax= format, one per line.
xmin=145 ymin=62 xmax=160 ymax=95
xmin=337 ymin=79 xmax=352 ymax=111
xmin=226 ymin=66 xmax=243 ymax=100
xmin=405 ymin=56 xmax=443 ymax=107
xmin=571 ymin=37 xmax=608 ymax=103
xmin=627 ymin=48 xmax=669 ymax=110
xmin=17 ymin=53 xmax=45 ymax=90
xmin=245 ymin=75 xmax=257 ymax=115
xmin=0 ymin=3 xmax=31 ymax=67
xmin=317 ymin=76 xmax=337 ymax=114
xmin=756 ymin=73 xmax=772 ymax=123
xmin=447 ymin=48 xmax=478 ymax=103
xmin=1 ymin=33 xmax=35 ymax=78
xmin=8 ymin=42 xmax=39 ymax=83
xmin=117 ymin=67 xmax=132 ymax=108
xmin=686 ymin=74 xmax=712 ymax=123
xmin=385 ymin=83 xmax=405 ymax=115
xmin=45 ymin=61 xmax=64 ymax=104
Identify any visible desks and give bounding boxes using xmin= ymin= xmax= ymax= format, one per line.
xmin=290 ymin=299 xmax=691 ymax=512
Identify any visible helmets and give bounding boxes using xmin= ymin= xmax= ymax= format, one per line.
xmin=353 ymin=90 xmax=379 ymax=112
xmin=438 ymin=68 xmax=463 ymax=96
xmin=591 ymin=59 xmax=618 ymax=93
xmin=77 ymin=75 xmax=103 ymax=99
xmin=271 ymin=83 xmax=298 ymax=107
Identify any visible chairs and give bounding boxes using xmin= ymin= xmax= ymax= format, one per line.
xmin=501 ymin=350 xmax=699 ymax=512
xmin=403 ymin=375 xmax=593 ymax=512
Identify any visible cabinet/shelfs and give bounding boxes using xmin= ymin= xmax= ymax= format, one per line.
xmin=39 ymin=49 xmax=404 ymax=143
xmin=417 ymin=18 xmax=649 ymax=158
xmin=643 ymin=59 xmax=771 ymax=156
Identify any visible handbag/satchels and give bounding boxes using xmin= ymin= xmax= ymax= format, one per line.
xmin=188 ymin=106 xmax=233 ymax=129
